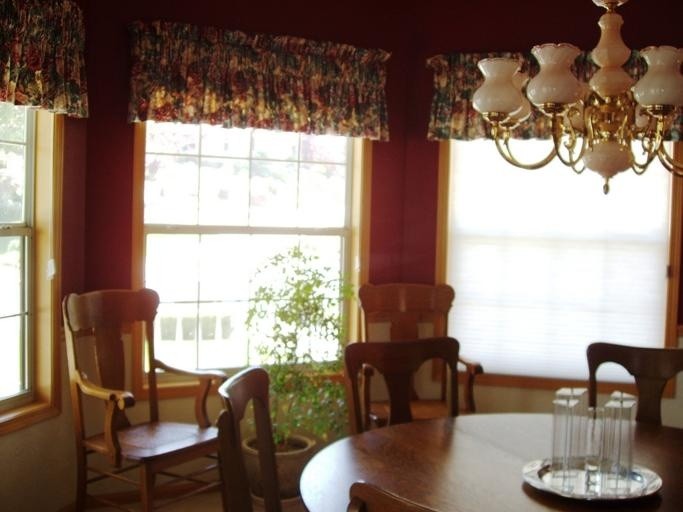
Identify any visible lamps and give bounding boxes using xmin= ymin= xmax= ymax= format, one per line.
xmin=468 ymin=1 xmax=683 ymax=195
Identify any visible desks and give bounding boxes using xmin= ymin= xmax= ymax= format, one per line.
xmin=296 ymin=410 xmax=682 ymax=511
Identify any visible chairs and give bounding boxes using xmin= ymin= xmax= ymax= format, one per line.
xmin=344 ymin=338 xmax=460 ymax=433
xmin=347 ymin=479 xmax=439 ymax=512
xmin=216 ymin=366 xmax=280 ymax=509
xmin=585 ymin=338 xmax=682 ymax=428
xmin=61 ymin=288 xmax=232 ymax=511
xmin=356 ymin=282 xmax=484 ymax=429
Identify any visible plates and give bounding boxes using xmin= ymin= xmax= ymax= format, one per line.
xmin=523 ymin=456 xmax=662 ymax=499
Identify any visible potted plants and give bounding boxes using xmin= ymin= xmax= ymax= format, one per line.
xmin=237 ymin=240 xmax=361 ymax=509
